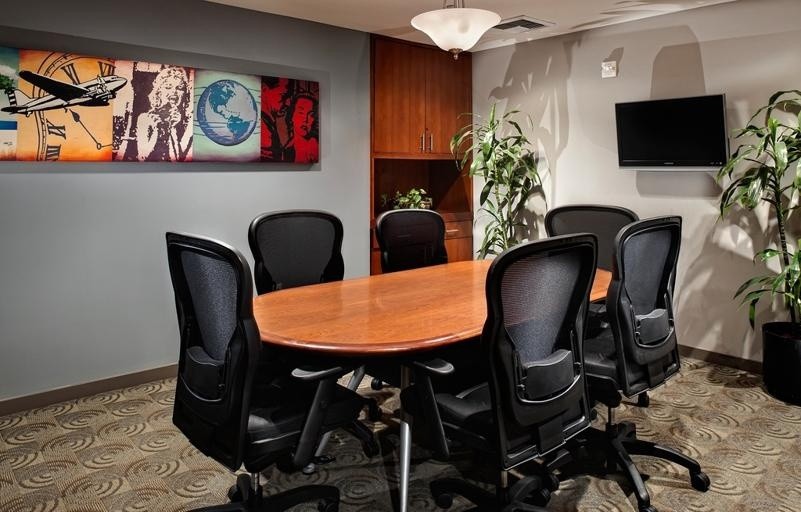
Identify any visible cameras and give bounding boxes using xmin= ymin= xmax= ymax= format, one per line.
xmin=154 ymin=102 xmax=172 ymax=120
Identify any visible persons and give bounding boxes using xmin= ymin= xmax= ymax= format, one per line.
xmin=283 ymin=90 xmax=320 ymax=165
xmin=259 ymin=75 xmax=298 ymax=164
xmin=135 ymin=66 xmax=195 ymax=162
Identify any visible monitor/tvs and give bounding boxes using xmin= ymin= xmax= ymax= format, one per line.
xmin=614 ymin=93 xmax=729 ymax=172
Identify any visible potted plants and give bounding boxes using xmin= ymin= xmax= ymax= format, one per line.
xmin=715 ymin=89 xmax=801 ymax=406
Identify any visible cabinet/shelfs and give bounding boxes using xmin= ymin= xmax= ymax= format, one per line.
xmin=371 ymin=33 xmax=475 ymax=270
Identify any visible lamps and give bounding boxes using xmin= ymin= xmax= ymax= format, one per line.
xmin=411 ymin=0 xmax=501 ymax=59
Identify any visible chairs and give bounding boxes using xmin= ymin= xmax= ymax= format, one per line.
xmin=165 ymin=202 xmax=710 ymax=512
